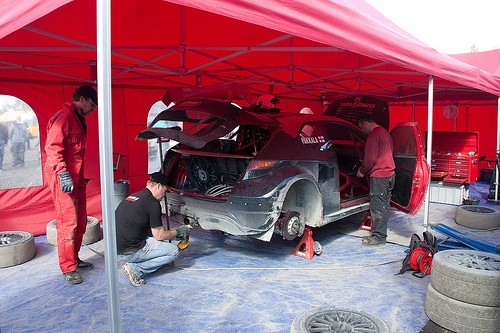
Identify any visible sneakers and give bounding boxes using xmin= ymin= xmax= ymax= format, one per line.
xmin=76 ymin=257 xmax=94 ymax=270
xmin=121 ymin=263 xmax=145 ymax=286
xmin=63 ymin=271 xmax=82 ymax=283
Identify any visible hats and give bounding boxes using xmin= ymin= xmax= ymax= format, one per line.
xmin=76 ymin=86 xmax=98 ymax=105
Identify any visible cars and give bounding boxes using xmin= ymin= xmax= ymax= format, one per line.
xmin=136 ymin=96 xmax=432 ymax=242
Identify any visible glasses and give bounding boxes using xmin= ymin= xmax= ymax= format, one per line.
xmin=83 ymin=95 xmax=96 ymax=109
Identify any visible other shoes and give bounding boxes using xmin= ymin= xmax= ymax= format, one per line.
xmin=363 ymin=236 xmax=385 ymax=246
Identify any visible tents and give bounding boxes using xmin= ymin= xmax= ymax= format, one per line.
xmin=0 ymin=0 xmax=500 ymax=333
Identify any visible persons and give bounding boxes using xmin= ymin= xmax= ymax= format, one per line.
xmin=356 ymin=115 xmax=396 ymax=246
xmin=115 ymin=172 xmax=190 ymax=286
xmin=42 ymin=84 xmax=99 ymax=283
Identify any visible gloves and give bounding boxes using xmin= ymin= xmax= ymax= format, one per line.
xmin=175 ymin=227 xmax=191 ymax=241
xmin=58 ymin=172 xmax=74 ymax=192
